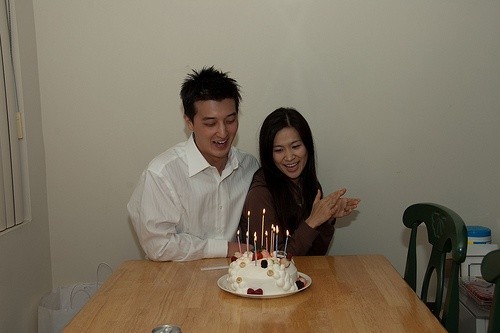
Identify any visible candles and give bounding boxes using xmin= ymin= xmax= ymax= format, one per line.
xmin=284 ymin=230 xmax=289 ymax=256
xmin=246 ymin=209 xmax=279 ymax=266
xmin=237 ymin=230 xmax=242 ymax=252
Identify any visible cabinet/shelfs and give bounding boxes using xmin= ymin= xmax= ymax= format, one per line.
xmin=443 ymin=278 xmax=490 ymax=333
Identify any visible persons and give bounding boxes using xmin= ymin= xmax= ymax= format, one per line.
xmin=239 ymin=104 xmax=361 ymax=257
xmin=125 ymin=67 xmax=262 ymax=262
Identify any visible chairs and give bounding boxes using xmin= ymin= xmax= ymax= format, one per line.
xmin=481 ymin=250 xmax=500 ymax=333
xmin=402 ymin=202 xmax=468 ymax=333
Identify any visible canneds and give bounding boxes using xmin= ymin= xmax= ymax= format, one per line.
xmin=151 ymin=324 xmax=182 ymax=333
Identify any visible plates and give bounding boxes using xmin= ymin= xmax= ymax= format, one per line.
xmin=217 ymin=271 xmax=312 ymax=299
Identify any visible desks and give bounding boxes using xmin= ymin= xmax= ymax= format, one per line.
xmin=60 ymin=254 xmax=451 ymax=333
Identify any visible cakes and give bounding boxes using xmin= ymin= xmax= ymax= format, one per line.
xmin=228 ymin=249 xmax=304 ymax=296
